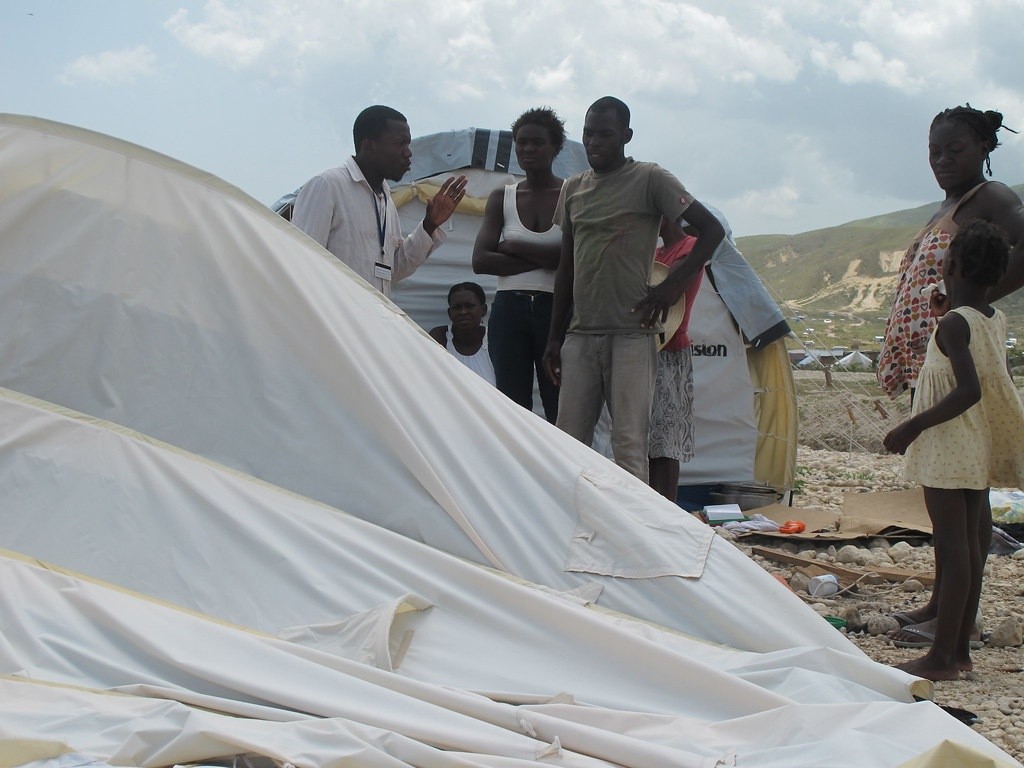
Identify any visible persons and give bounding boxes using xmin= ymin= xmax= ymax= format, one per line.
xmin=882 ymin=220 xmax=1024 ymax=682
xmin=541 ymin=96 xmax=726 ymax=487
xmin=646 ymin=213 xmax=704 ymax=504
xmin=879 ymin=101 xmax=1024 ymax=647
xmin=429 ymin=281 xmax=496 ymax=390
xmin=469 ymin=108 xmax=568 ymax=427
xmin=288 ymin=106 xmax=467 ymax=301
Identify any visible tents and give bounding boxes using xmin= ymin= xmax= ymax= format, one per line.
xmin=268 ymin=126 xmax=801 ymax=511
xmin=0 ymin=113 xmax=1024 ymax=768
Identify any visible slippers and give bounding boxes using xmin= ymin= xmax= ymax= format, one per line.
xmin=884 ymin=612 xmax=917 ymax=628
xmin=883 ymin=625 xmax=981 ymax=649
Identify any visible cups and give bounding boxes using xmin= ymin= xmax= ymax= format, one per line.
xmin=808 ymin=574 xmax=839 ymax=597
xmin=823 ymin=616 xmax=846 ymax=631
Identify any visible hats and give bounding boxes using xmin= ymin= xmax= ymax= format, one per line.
xmin=647 ymin=261 xmax=685 ymax=353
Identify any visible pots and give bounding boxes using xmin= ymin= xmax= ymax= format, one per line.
xmin=707 ymin=480 xmax=784 ymax=511
